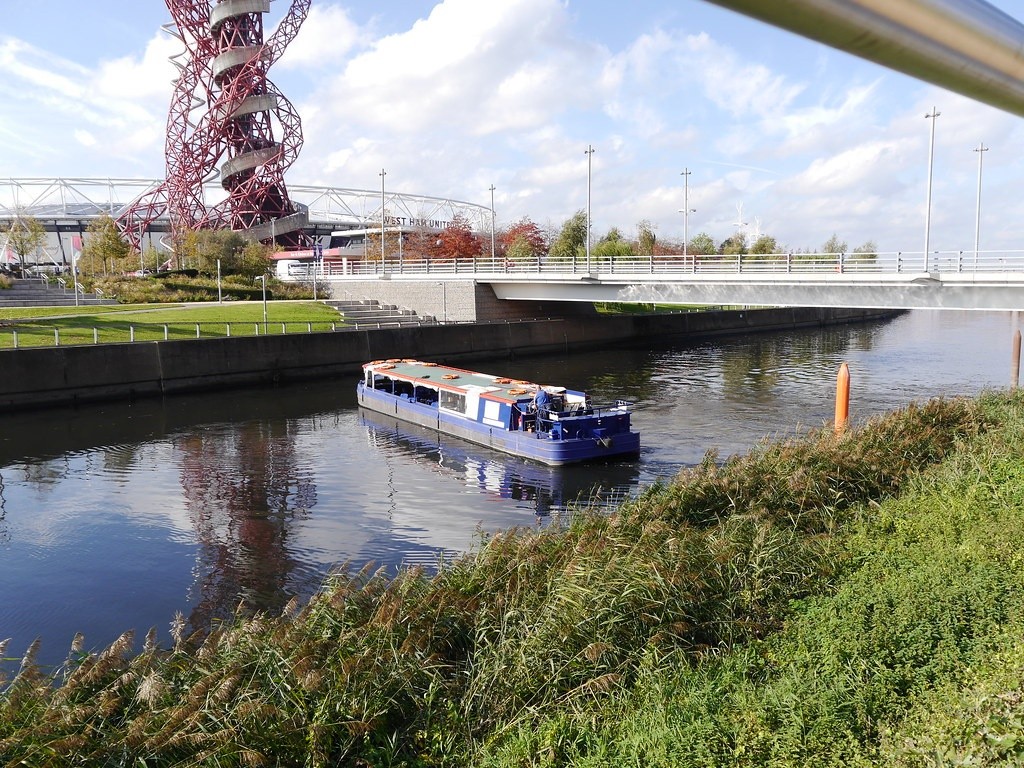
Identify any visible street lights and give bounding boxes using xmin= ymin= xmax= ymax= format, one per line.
xmin=256 ymin=275 xmax=268 ymax=334
xmin=678 ymin=208 xmax=696 ymax=252
xmin=681 ymin=171 xmax=691 ymax=272
xmin=61 ymin=238 xmax=67 ymax=269
xmin=973 ymin=147 xmax=988 ymax=269
xmin=380 ymin=172 xmax=386 ymax=274
xmin=924 ymin=113 xmax=942 ymax=272
xmin=437 ymin=282 xmax=447 ymax=325
xmin=585 ymin=149 xmax=595 ymax=273
xmin=489 ymin=187 xmax=495 ymax=273
xmin=733 ymin=223 xmax=749 ymax=236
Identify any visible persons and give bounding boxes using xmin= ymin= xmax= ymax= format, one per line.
xmin=534 ymin=385 xmax=550 ymax=432
xmin=66 ymin=264 xmax=71 ymax=275
xmin=55 ymin=265 xmax=59 ymax=276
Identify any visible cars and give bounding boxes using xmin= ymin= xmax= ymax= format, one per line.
xmin=136 ymin=270 xmax=149 ymax=276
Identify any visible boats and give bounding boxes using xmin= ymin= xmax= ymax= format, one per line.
xmin=357 ymin=358 xmax=641 ymax=465
